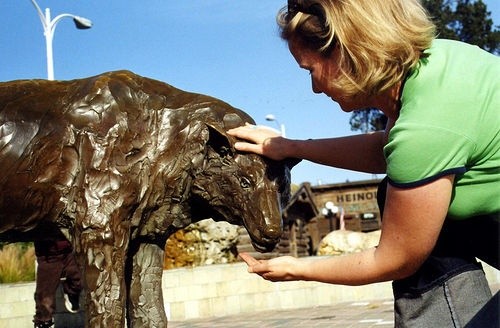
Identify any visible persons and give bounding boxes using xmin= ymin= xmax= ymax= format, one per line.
xmin=5 ymin=223 xmax=85 ymax=328
xmin=225 ymin=0 xmax=500 ymax=328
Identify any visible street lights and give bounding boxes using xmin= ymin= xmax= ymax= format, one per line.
xmin=321 ymin=201 xmax=339 ymax=233
xmin=29 ymin=0 xmax=93 ymax=81
